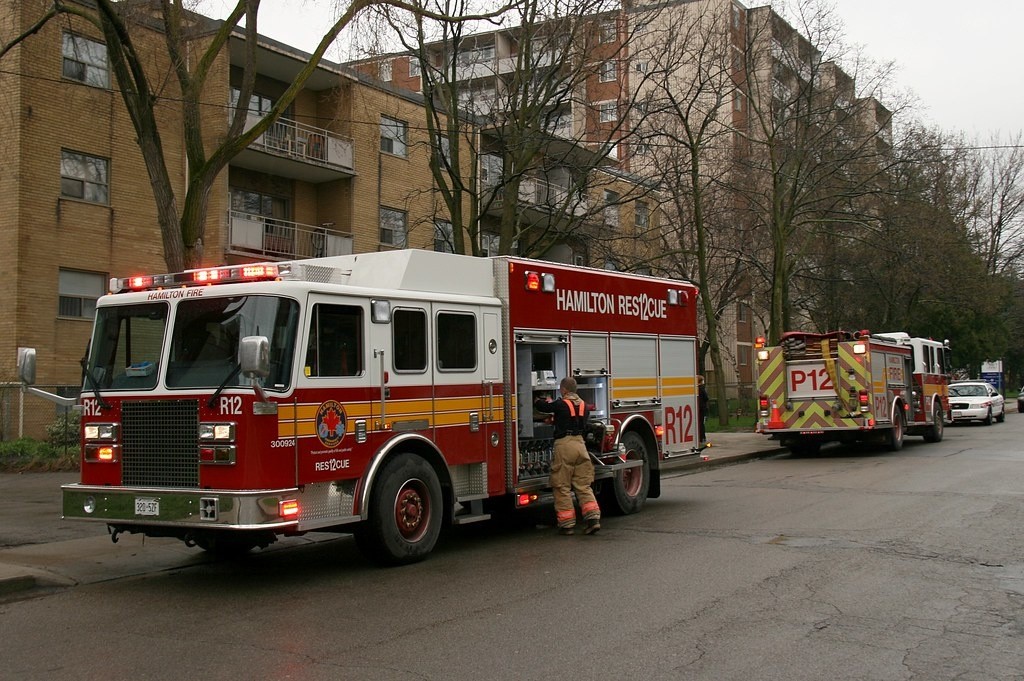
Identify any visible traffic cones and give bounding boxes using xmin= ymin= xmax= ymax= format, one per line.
xmin=766 ymin=401 xmax=786 ymax=430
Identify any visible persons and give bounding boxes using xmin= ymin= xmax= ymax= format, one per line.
xmin=532 ymin=378 xmax=601 ymax=536
xmin=698 ymin=376 xmax=708 ymax=443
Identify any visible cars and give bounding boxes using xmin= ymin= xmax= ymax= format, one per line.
xmin=1017 ymin=387 xmax=1024 ymax=412
xmin=947 ymin=382 xmax=1006 ymax=427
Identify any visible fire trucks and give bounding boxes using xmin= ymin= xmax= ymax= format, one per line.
xmin=755 ymin=331 xmax=953 ymax=453
xmin=16 ymin=247 xmax=714 ymax=567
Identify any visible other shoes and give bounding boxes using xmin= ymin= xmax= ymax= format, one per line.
xmin=558 ymin=528 xmax=575 ymax=535
xmin=583 ymin=518 xmax=600 ymax=535
